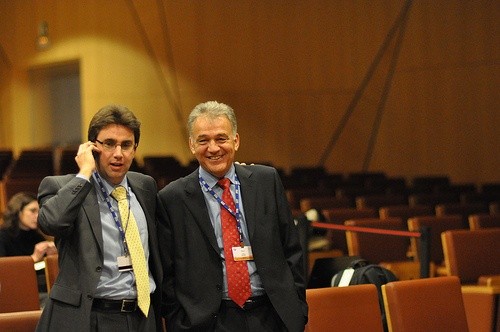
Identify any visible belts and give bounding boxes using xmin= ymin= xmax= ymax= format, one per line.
xmin=221 ymin=295 xmax=270 ymax=309
xmin=92 ymin=298 xmax=140 ymax=313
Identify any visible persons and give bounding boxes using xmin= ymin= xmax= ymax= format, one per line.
xmin=36 ymin=106 xmax=162 ymax=332
xmin=0 ymin=193 xmax=57 ymax=262
xmin=157 ymin=101 xmax=308 ymax=332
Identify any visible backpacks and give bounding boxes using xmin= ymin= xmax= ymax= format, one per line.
xmin=330 ymin=259 xmax=400 ymax=332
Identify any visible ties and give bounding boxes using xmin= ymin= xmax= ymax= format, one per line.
xmin=217 ymin=178 xmax=252 ymax=307
xmin=110 ymin=185 xmax=151 ymax=318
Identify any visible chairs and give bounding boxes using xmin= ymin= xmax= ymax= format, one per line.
xmin=128 ymin=154 xmax=199 ymax=193
xmin=274 ymin=166 xmax=500 ymax=332
xmin=0 ymin=147 xmax=80 ymax=332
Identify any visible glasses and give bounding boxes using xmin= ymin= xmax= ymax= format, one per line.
xmin=96 ymin=139 xmax=138 ymax=151
